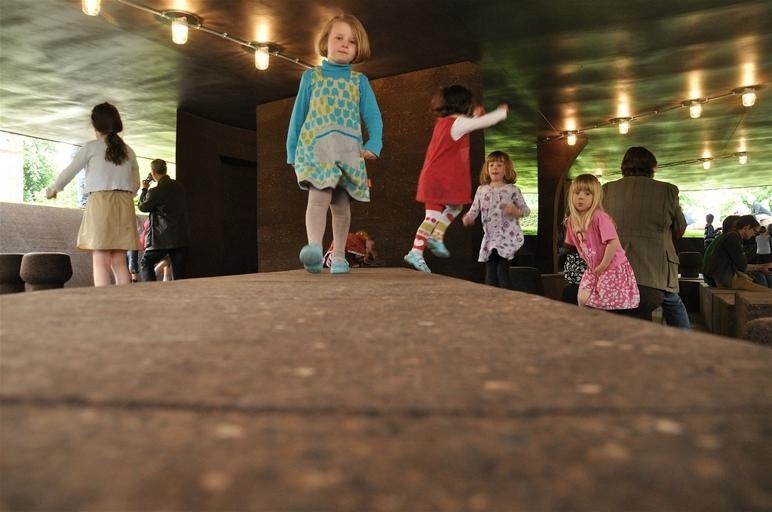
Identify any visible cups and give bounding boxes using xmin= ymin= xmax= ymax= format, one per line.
xmin=144 ymin=173 xmax=153 ymax=183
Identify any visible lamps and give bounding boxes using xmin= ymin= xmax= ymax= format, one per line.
xmin=81 ymin=0 xmax=102 ymax=17
xmin=700 ymin=157 xmax=713 ymax=170
xmin=170 ymin=16 xmax=189 ymax=45
xmin=741 ymin=85 xmax=756 ymax=107
xmin=618 ymin=117 xmax=630 ymax=135
xmin=566 ymin=131 xmax=577 ymax=146
xmin=737 ymin=151 xmax=748 ymax=165
xmin=254 ymin=45 xmax=270 ymax=71
xmin=688 ymin=100 xmax=702 ymax=119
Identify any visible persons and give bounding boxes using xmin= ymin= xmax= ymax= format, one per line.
xmin=139 ymin=158 xmax=192 ymax=282
xmin=403 ymin=85 xmax=511 ymax=273
xmin=139 ymin=217 xmax=172 ymax=282
xmin=285 ymin=14 xmax=384 ymax=275
xmin=461 ymin=151 xmax=530 ymax=289
xmin=563 ymin=173 xmax=640 ymax=311
xmin=755 ymin=226 xmax=772 ymax=264
xmin=554 ymin=190 xmax=587 ymax=287
xmin=702 ymin=215 xmax=769 ymax=288
xmin=711 ymin=215 xmax=772 ymax=291
xmin=602 ymin=146 xmax=691 ymax=330
xmin=322 ymin=230 xmax=377 ymax=269
xmin=704 ymin=214 xmax=723 ymax=252
xmin=45 ymin=101 xmax=142 ymax=287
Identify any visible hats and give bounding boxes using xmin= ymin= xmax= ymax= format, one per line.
xmin=324 ymin=232 xmax=374 ymax=267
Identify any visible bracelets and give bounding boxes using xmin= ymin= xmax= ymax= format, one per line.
xmin=142 ymin=188 xmax=147 ymax=192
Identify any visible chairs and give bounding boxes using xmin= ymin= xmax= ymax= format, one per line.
xmin=508 ymin=266 xmax=545 ymax=297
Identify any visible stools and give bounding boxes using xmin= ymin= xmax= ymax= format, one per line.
xmin=698 ymin=281 xmax=772 ymax=345
xmin=0 ymin=253 xmax=24 ymax=294
xmin=20 ymin=252 xmax=73 ymax=292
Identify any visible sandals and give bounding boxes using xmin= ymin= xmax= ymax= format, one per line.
xmin=426 ymin=238 xmax=450 ymax=258
xmin=299 ymin=244 xmax=324 ymax=274
xmin=331 ymin=257 xmax=350 ymax=273
xmin=404 ymin=252 xmax=431 ymax=274
